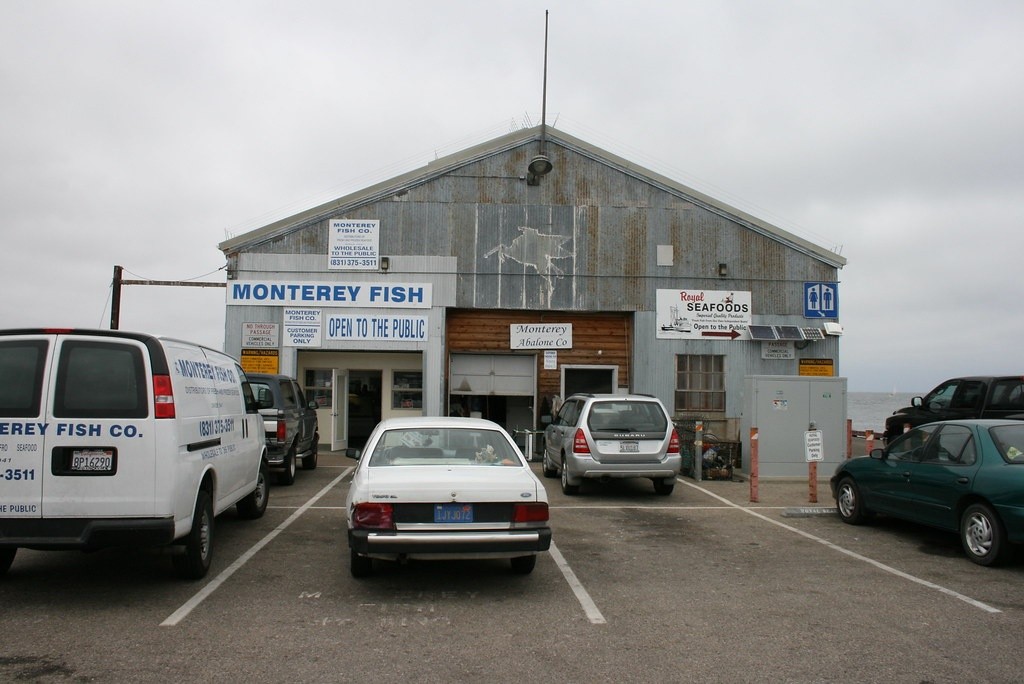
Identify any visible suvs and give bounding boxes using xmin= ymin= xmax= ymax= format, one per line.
xmin=240 ymin=374 xmax=320 ymax=486
xmin=882 ymin=376 xmax=1024 ymax=445
xmin=540 ymin=392 xmax=682 ymax=496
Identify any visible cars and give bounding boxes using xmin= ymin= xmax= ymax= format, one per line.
xmin=830 ymin=418 xmax=1024 ymax=566
xmin=345 ymin=417 xmax=552 ymax=579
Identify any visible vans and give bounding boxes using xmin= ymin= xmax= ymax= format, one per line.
xmin=0 ymin=328 xmax=272 ymax=581
